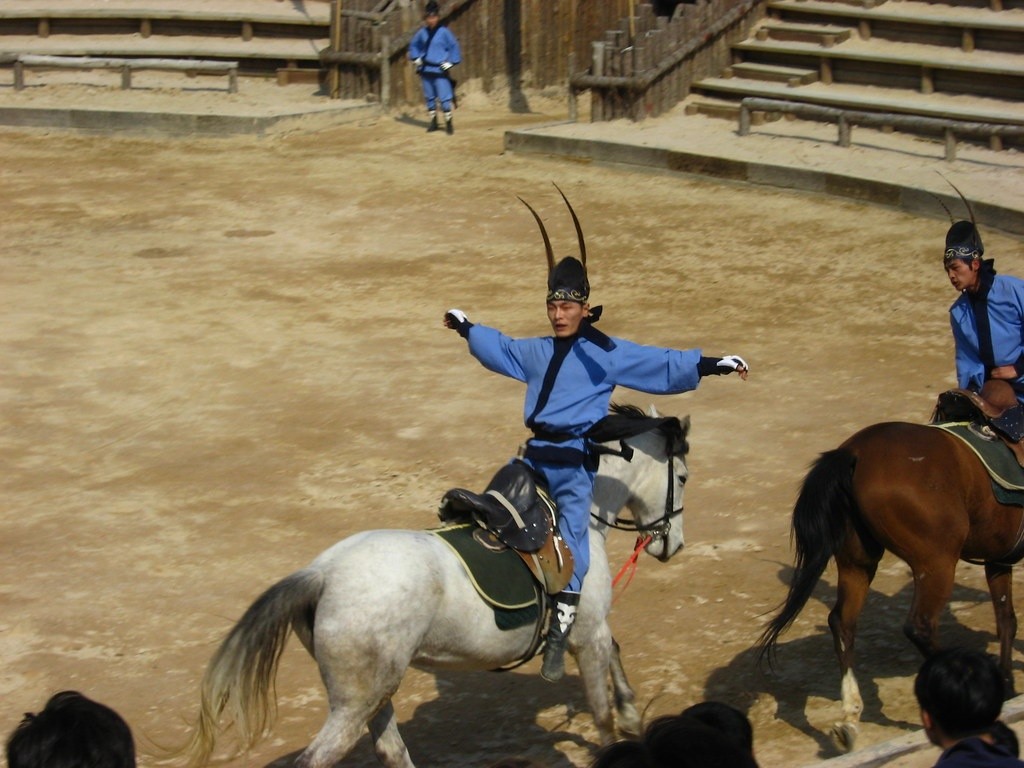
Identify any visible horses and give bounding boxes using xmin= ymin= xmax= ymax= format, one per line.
xmin=134 ymin=400 xmax=691 ymax=768
xmin=747 ymin=379 xmax=1024 ymax=754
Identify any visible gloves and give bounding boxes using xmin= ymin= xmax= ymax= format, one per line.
xmin=440 ymin=62 xmax=453 ymax=70
xmin=412 ymin=58 xmax=423 ymax=69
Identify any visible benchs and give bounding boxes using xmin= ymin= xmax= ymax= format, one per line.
xmin=0 ymin=0 xmax=334 ymax=76
xmin=687 ymin=0 xmax=1024 ymax=147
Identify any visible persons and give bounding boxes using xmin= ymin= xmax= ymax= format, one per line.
xmin=409 ymin=0 xmax=462 ymax=135
xmin=5 ymin=689 xmax=137 ymax=768
xmin=941 ymin=219 xmax=1024 ymax=422
xmin=441 ymin=256 xmax=751 ymax=683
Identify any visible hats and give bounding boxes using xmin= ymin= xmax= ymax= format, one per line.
xmin=426 ymin=0 xmax=439 ymax=16
xmin=929 ymin=171 xmax=984 ymax=264
xmin=517 ymin=181 xmax=590 ymax=305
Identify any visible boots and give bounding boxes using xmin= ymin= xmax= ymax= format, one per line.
xmin=443 ymin=110 xmax=454 ymax=135
xmin=427 ymin=109 xmax=438 ymax=131
xmin=540 ymin=590 xmax=581 ymax=683
